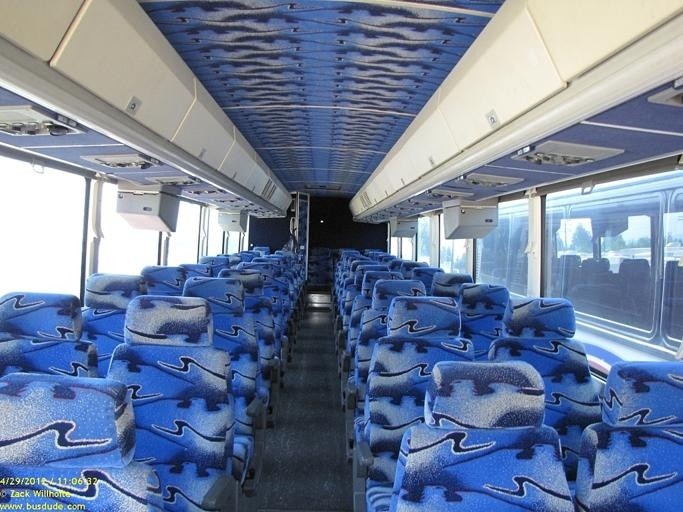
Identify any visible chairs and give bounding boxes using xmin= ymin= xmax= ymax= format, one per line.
xmin=333 ymin=248 xmax=683 ymax=512
xmin=0 ymin=245 xmax=304 ymax=512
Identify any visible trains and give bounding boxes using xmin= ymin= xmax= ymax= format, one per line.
xmin=404 ymin=173 xmax=683 ymax=407
